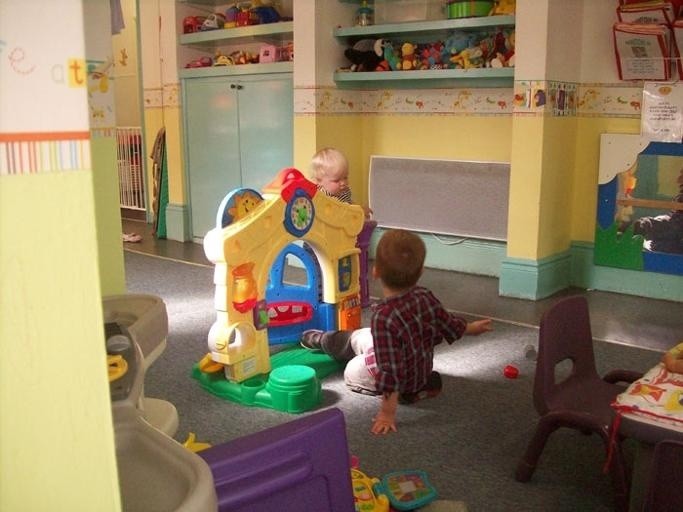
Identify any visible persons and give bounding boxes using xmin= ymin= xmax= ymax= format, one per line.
xmin=311 ymin=147 xmax=352 ymax=205
xmin=299 ymin=229 xmax=494 ymax=436
xmin=632 ymin=168 xmax=683 ymax=255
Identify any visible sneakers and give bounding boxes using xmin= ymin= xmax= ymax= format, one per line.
xmin=122 ymin=231 xmax=144 ymax=243
xmin=299 ymin=329 xmax=322 ymax=350
xmin=397 ymin=371 xmax=443 ymax=405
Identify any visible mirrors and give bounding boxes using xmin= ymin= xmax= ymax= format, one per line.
xmin=594 ymin=131 xmax=683 ymax=279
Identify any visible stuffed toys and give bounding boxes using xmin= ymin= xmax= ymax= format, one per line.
xmin=343 ymin=29 xmax=514 ymax=71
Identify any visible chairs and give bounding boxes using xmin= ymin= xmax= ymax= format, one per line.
xmin=516 ymin=294 xmax=649 ymax=511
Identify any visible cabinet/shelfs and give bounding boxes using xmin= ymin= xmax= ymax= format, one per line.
xmin=175 ymin=21 xmax=293 ymax=79
xmin=330 ymin=13 xmax=517 ymax=87
xmin=179 ymin=73 xmax=292 ymax=247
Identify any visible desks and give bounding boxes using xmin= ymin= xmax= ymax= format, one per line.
xmin=612 ymin=344 xmax=683 ymax=446
